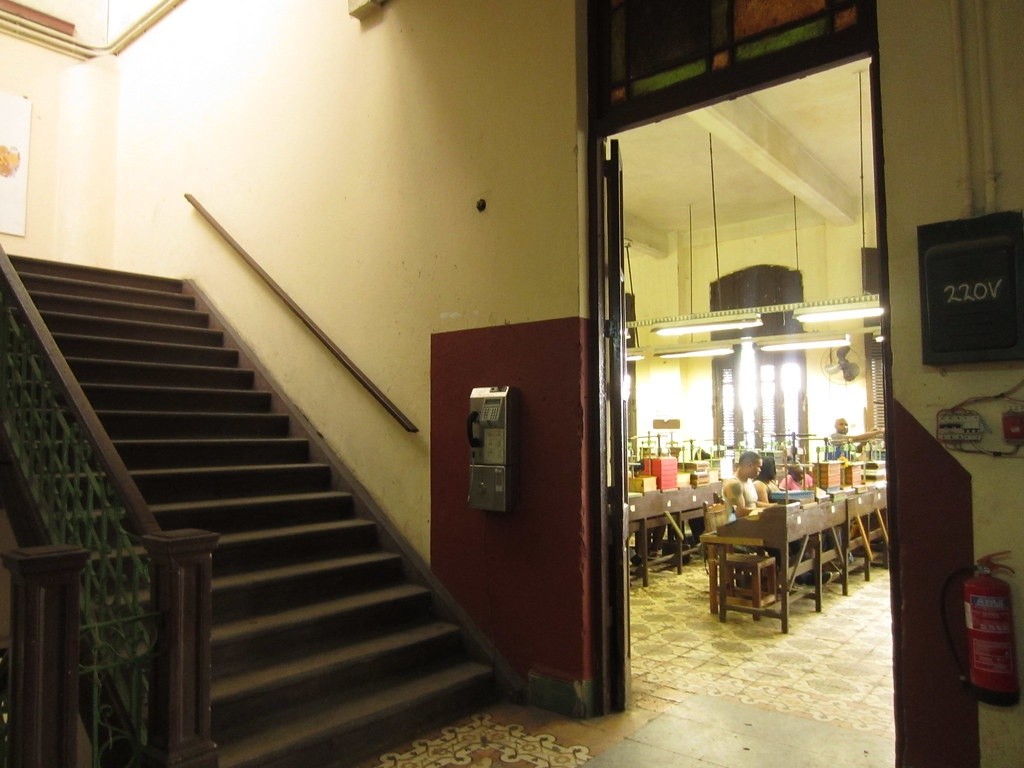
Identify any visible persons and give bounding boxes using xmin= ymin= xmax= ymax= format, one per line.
xmin=723 ymin=451 xmax=853 ymax=586
xmin=825 ymin=418 xmax=885 ymax=461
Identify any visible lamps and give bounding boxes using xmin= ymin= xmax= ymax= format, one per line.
xmin=626 ymin=70 xmax=886 ymax=361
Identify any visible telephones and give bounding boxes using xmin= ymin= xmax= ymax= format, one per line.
xmin=467 ymin=385 xmax=519 ymax=512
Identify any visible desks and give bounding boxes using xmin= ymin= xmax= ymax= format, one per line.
xmin=629 ymin=459 xmax=886 ymax=633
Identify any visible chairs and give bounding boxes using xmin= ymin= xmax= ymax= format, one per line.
xmin=703 ymin=498 xmax=778 ymax=622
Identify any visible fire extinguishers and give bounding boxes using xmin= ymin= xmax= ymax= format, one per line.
xmin=938 ymin=549 xmax=1020 ymax=707
